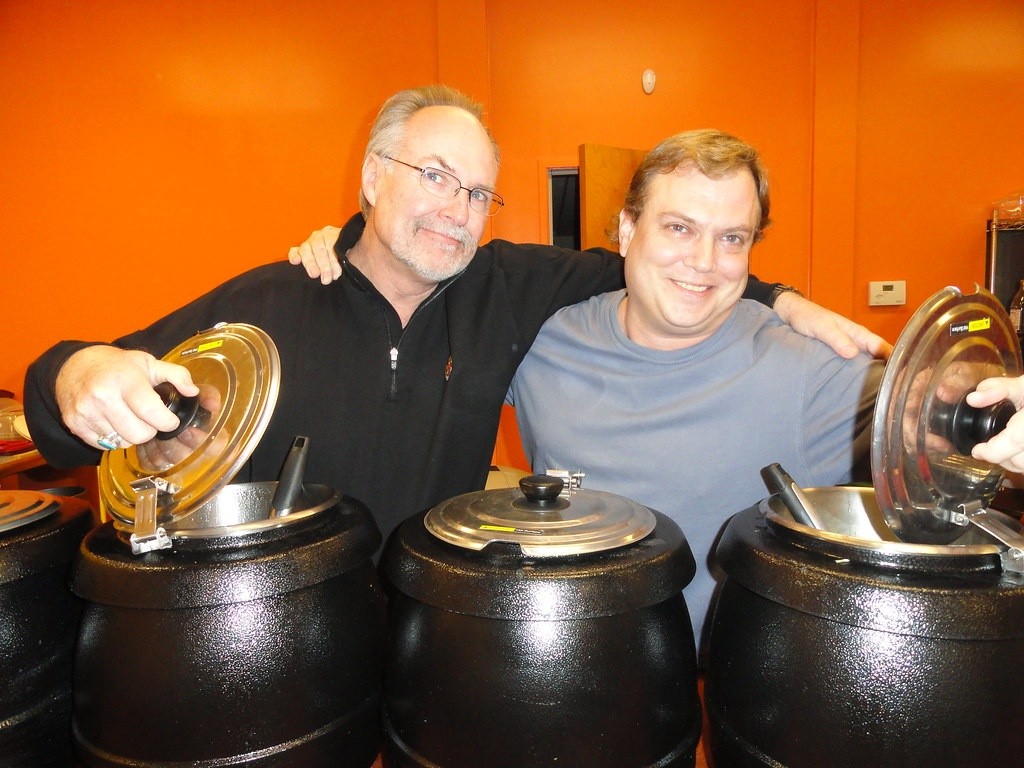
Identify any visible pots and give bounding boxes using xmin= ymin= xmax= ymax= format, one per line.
xmin=699 ymin=282 xmax=1024 ymax=768
xmin=0 ymin=489 xmax=95 ymax=768
xmin=378 ymin=472 xmax=699 ymax=768
xmin=76 ymin=322 xmax=382 ymax=768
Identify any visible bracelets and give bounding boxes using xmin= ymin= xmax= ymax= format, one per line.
xmin=768 ymin=284 xmax=805 ymax=309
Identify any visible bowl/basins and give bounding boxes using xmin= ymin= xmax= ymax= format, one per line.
xmin=0 ymin=397 xmax=27 ymax=441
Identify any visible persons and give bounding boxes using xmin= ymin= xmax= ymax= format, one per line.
xmin=22 ymin=85 xmax=893 ymax=576
xmin=887 ymin=360 xmax=1024 ymax=490
xmin=287 ymin=129 xmax=886 ymax=671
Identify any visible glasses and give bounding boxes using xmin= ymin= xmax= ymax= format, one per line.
xmin=385 ymin=156 xmax=504 ymax=217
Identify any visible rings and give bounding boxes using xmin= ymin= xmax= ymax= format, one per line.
xmin=98 ymin=432 xmax=123 ymax=450
